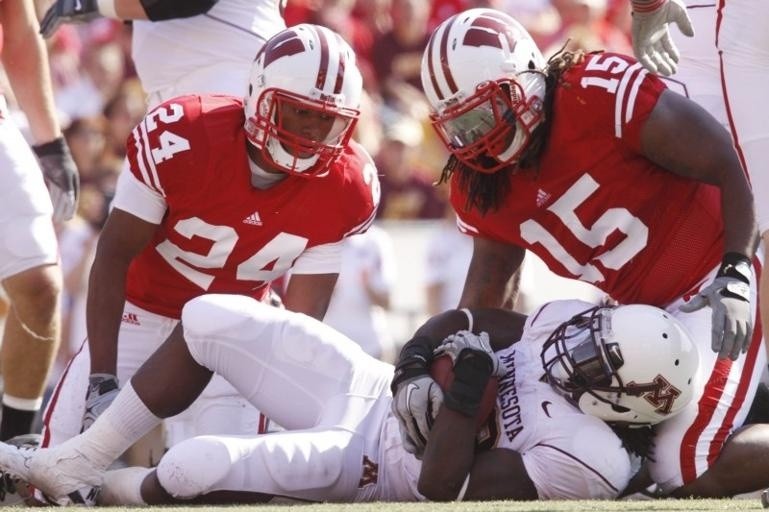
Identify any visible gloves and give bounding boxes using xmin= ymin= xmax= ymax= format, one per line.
xmin=81 ymin=371 xmax=121 ymax=434
xmin=631 ymin=0 xmax=693 ymax=78
xmin=433 ymin=328 xmax=508 ymax=419
xmin=678 ymin=252 xmax=753 ymax=361
xmin=40 ymin=0 xmax=105 ymax=40
xmin=390 ymin=337 xmax=444 ymax=454
xmin=32 ymin=134 xmax=81 ymax=224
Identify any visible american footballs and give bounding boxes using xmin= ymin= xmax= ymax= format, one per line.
xmin=431 ymin=353 xmax=498 ymax=437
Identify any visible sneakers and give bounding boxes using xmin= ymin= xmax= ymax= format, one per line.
xmin=1 ymin=434 xmax=102 ymax=508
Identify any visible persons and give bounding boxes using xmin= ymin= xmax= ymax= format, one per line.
xmin=417 ymin=3 xmax=769 ymax=500
xmin=0 ymin=2 xmax=81 ymax=501
xmin=1 ymin=0 xmax=767 ymax=435
xmin=0 ymin=292 xmax=699 ymax=506
xmin=8 ymin=21 xmax=382 ymax=508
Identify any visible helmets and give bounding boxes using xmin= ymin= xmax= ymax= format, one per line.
xmin=540 ymin=304 xmax=699 ymax=433
xmin=419 ymin=8 xmax=550 ymax=174
xmin=243 ymin=22 xmax=364 ymax=182
